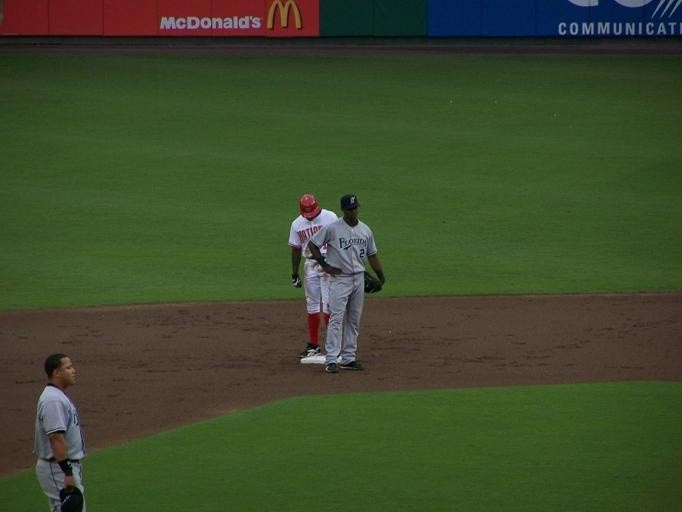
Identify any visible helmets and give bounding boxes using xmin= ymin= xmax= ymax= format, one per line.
xmin=299 ymin=194 xmax=320 ymax=219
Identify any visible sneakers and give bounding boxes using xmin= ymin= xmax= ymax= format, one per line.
xmin=325 ymin=360 xmax=363 ymax=372
xmin=298 ymin=342 xmax=322 ymax=357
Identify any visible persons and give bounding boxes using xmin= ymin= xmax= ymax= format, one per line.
xmin=288 ymin=193 xmax=385 ymax=374
xmin=33 ymin=353 xmax=87 ymax=512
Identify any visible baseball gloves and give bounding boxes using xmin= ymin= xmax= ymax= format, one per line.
xmin=364 ymin=272 xmax=382 ymax=293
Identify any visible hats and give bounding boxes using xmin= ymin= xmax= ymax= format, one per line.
xmin=341 ymin=194 xmax=360 ymax=209
xmin=60 ymin=485 xmax=83 ymax=512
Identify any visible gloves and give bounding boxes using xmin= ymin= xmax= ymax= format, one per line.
xmin=291 ymin=274 xmax=302 ymax=288
